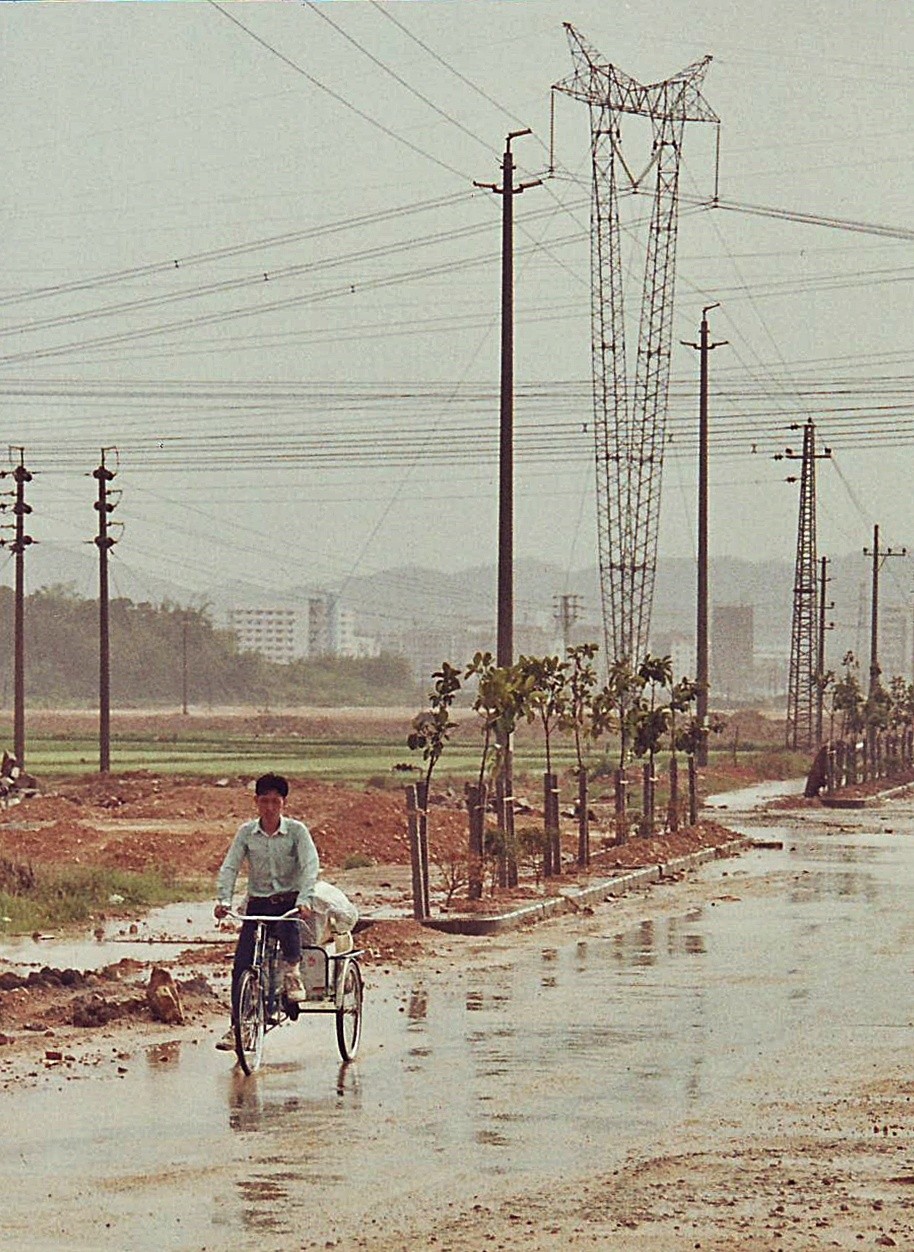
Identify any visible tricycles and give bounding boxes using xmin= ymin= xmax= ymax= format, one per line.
xmin=211 ymin=899 xmax=364 ymax=1077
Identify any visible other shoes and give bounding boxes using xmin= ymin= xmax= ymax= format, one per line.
xmin=214 ymin=1029 xmax=248 ymax=1051
xmin=283 ymin=972 xmax=305 ymax=1002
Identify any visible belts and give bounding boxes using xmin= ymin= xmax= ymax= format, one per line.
xmin=247 ymin=891 xmax=299 ymax=907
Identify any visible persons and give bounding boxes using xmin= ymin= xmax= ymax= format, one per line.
xmin=213 ymin=772 xmax=320 ymax=1051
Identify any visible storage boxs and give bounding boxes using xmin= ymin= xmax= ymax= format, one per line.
xmin=272 ymin=909 xmax=339 ymax=987
